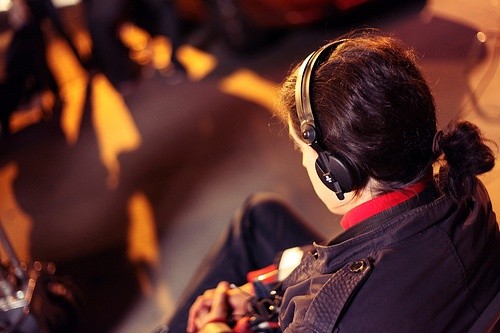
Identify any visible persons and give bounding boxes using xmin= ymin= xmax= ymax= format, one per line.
xmin=160 ymin=31 xmax=500 ymax=333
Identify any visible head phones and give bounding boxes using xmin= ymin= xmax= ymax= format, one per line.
xmin=295 ymin=39 xmax=366 ymax=200
